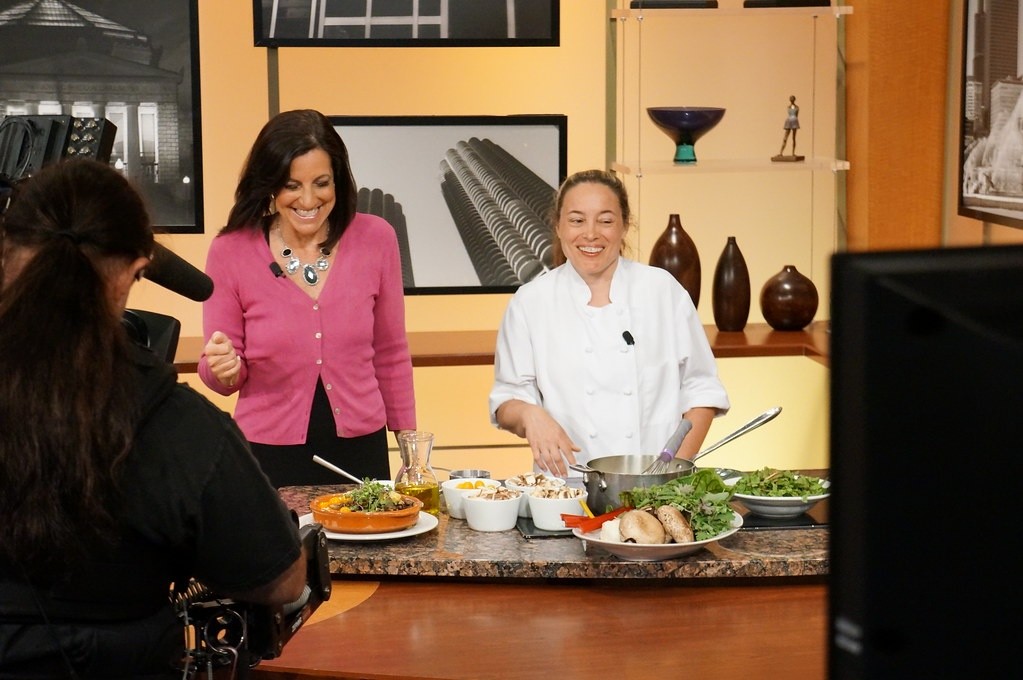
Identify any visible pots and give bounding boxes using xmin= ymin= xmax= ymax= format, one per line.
xmin=568 ymin=406 xmax=783 ymax=518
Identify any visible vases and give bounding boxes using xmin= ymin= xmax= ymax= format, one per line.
xmin=648 ymin=214 xmax=700 ymax=311
xmin=712 ymin=236 xmax=751 ymax=334
xmin=761 ymin=264 xmax=818 ymax=332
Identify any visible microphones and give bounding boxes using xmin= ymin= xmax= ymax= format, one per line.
xmin=623 ymin=331 xmax=635 ymax=346
xmin=143 ymin=241 xmax=214 ymax=302
xmin=270 ymin=261 xmax=283 ymax=278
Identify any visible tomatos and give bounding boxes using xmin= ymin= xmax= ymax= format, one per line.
xmin=318 ymin=494 xmax=351 ymax=513
xmin=455 ymin=480 xmax=486 ymax=489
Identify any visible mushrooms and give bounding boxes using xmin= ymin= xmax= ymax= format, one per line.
xmin=619 ymin=509 xmax=666 ymax=544
xmin=468 ymin=472 xmax=584 ymax=500
xmin=657 ymin=505 xmax=695 ymax=543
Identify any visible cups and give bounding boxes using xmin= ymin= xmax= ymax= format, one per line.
xmin=448 ymin=469 xmax=490 ymax=480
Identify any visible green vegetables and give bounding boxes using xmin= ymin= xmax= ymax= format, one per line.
xmin=729 ymin=466 xmax=830 ymax=504
xmin=559 ymin=468 xmax=736 ymax=542
xmin=345 ymin=476 xmax=395 ymax=513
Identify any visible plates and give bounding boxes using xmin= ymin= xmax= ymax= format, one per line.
xmin=723 ymin=475 xmax=831 ymax=518
xmin=299 ymin=511 xmax=439 ymax=541
xmin=572 ymin=509 xmax=743 ymax=562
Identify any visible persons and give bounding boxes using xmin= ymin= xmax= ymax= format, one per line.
xmin=198 ymin=109 xmax=416 ymax=487
xmin=489 ymin=170 xmax=730 ymax=478
xmin=778 ymin=95 xmax=800 ymax=157
xmin=0 ymin=159 xmax=306 ymax=680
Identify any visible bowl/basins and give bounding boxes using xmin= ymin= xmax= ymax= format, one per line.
xmin=504 ymin=475 xmax=566 ymax=518
xmin=461 ymin=489 xmax=523 ymax=532
xmin=309 ymin=491 xmax=422 ymax=534
xmin=441 ymin=478 xmax=502 ymax=519
xmin=646 ymin=106 xmax=726 ymax=163
xmin=527 ymin=488 xmax=588 ymax=532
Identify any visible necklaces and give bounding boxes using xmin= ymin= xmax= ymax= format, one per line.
xmin=277 ymin=216 xmax=331 ymax=286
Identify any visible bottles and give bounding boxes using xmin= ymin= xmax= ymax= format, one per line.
xmin=758 ymin=264 xmax=819 ymax=333
xmin=648 ymin=213 xmax=702 ymax=310
xmin=712 ymin=236 xmax=752 ymax=333
xmin=395 ymin=431 xmax=441 ymax=517
